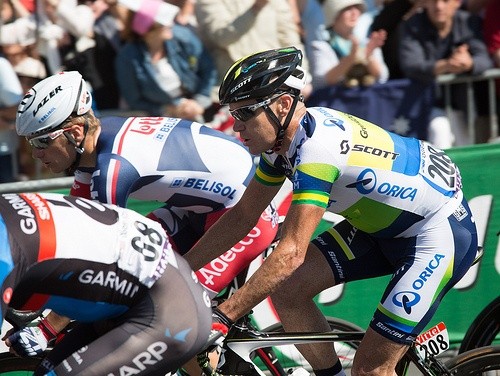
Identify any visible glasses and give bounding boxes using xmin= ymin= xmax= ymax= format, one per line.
xmin=26 ymin=124 xmax=84 ymax=150
xmin=228 ymin=92 xmax=296 ymax=124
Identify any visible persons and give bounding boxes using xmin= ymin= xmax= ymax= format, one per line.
xmin=0 ymin=0 xmax=500 ymax=184
xmin=0 ymin=192 xmax=212 ymax=376
xmin=310 ymin=0 xmax=389 ymax=133
xmin=16 ymin=71 xmax=279 ymax=376
xmin=181 ymin=47 xmax=478 ymax=376
xmin=394 ymin=0 xmax=491 ymax=151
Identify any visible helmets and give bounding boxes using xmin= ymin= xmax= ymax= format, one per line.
xmin=15 ymin=71 xmax=92 ymax=136
xmin=218 ymin=46 xmax=307 ymax=105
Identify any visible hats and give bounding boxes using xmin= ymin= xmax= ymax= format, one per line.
xmin=323 ymin=0 xmax=368 ymax=30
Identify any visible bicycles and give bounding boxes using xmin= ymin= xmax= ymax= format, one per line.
xmin=0 ymin=296 xmax=500 ymax=376
